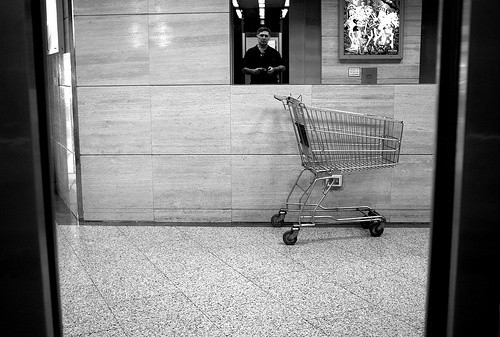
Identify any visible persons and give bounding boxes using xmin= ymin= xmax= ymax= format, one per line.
xmin=241 ymin=27 xmax=286 ymax=84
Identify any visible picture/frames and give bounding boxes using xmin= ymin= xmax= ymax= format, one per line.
xmin=337 ymin=0 xmax=405 ymax=61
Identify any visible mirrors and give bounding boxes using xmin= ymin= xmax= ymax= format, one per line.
xmin=229 ymin=0 xmax=440 ymax=86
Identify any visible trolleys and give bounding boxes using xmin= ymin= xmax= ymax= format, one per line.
xmin=270 ymin=93 xmax=404 ymax=245
